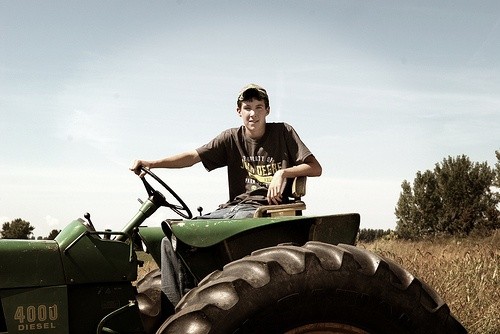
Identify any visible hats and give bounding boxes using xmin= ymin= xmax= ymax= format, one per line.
xmin=235 ymin=82 xmax=267 ymax=100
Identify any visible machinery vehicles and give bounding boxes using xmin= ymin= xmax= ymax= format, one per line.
xmin=0 ymin=166 xmax=474 ymax=333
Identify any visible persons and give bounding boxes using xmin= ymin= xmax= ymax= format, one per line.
xmin=129 ymin=87 xmax=323 ymax=317
